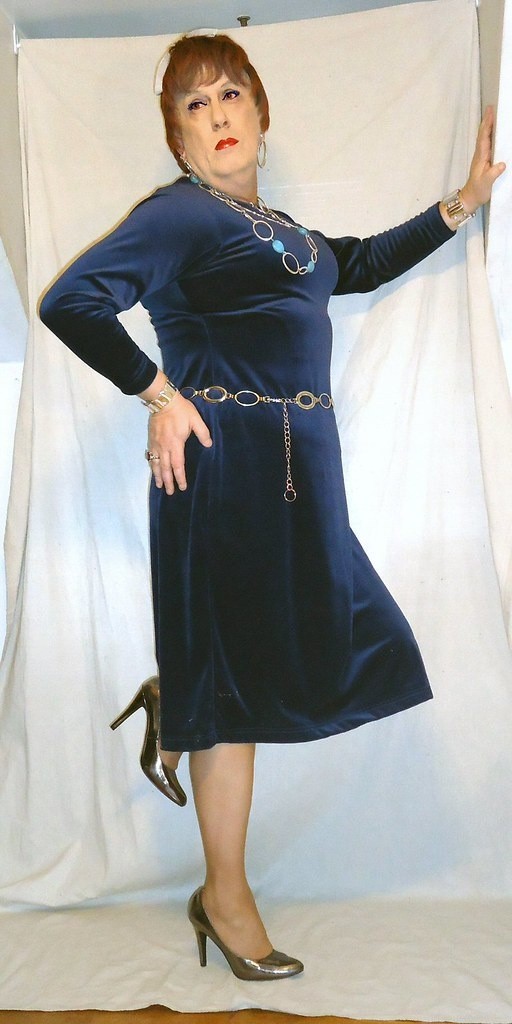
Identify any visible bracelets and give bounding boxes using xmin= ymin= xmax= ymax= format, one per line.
xmin=441 ymin=188 xmax=476 ymax=226
xmin=141 ymin=381 xmax=177 ymax=415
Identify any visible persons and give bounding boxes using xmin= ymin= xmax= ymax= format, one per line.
xmin=36 ymin=29 xmax=507 ymax=982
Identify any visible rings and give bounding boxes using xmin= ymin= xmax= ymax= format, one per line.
xmin=143 ymin=449 xmax=160 ymax=463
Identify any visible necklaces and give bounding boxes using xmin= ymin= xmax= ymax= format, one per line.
xmin=185 ymin=173 xmax=321 ymax=274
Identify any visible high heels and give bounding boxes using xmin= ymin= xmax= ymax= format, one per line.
xmin=109 ymin=674 xmax=187 ymax=808
xmin=186 ymin=885 xmax=305 ymax=982
